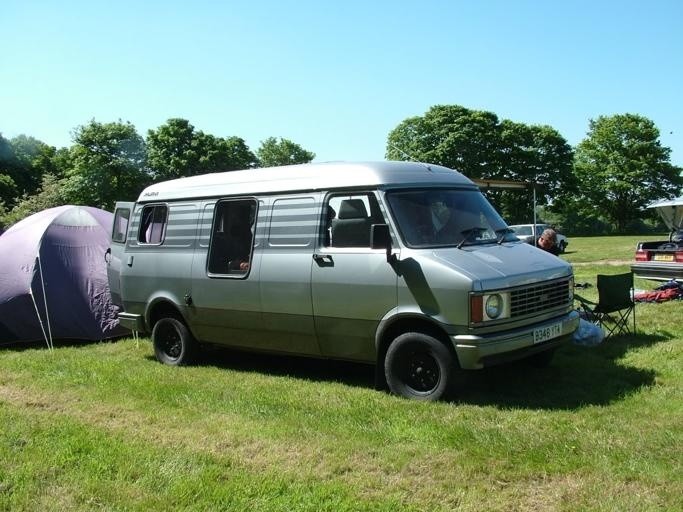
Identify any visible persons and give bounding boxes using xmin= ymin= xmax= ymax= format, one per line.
xmin=522 ymin=228 xmax=559 ymax=257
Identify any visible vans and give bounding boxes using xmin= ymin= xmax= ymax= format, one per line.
xmin=102 ymin=157 xmax=578 ymax=407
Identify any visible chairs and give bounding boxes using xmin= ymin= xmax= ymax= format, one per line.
xmin=573 ymin=270 xmax=642 ymax=342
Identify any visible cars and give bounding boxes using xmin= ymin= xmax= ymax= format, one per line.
xmin=509 ymin=224 xmax=569 ymax=254
xmin=630 ymin=199 xmax=683 ymax=282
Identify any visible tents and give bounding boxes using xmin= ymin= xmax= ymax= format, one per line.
xmin=0 ymin=204 xmax=167 ymax=349
